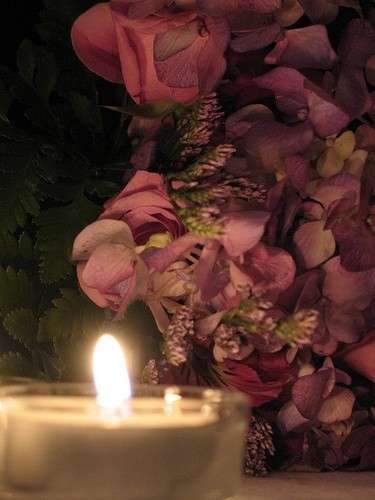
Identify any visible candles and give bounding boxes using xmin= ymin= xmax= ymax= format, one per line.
xmin=1 ymin=334 xmax=251 ymax=500
